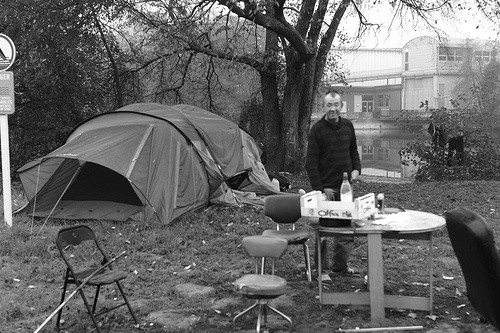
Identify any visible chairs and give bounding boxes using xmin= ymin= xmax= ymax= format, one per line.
xmin=57 ymin=225 xmax=138 ymax=333
xmin=262 ymin=195 xmax=312 ymax=282
xmin=233 ymin=235 xmax=293 ymax=333
xmin=445 ymin=206 xmax=500 ymax=328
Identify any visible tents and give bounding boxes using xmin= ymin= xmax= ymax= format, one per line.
xmin=9 ymin=103 xmax=282 ymax=229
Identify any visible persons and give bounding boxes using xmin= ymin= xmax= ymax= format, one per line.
xmin=305 ymin=90 xmax=362 ymax=285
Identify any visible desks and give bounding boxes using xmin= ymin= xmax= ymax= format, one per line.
xmin=318 ymin=210 xmax=445 ymax=332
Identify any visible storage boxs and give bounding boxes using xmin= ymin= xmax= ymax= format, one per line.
xmin=301 ymin=190 xmax=375 ymax=220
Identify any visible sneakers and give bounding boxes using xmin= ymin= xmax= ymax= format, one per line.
xmin=317 ymin=274 xmax=332 ymax=284
xmin=331 ymin=267 xmax=360 ymax=277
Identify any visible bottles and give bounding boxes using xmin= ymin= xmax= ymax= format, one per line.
xmin=340 ymin=173 xmax=353 ymax=202
